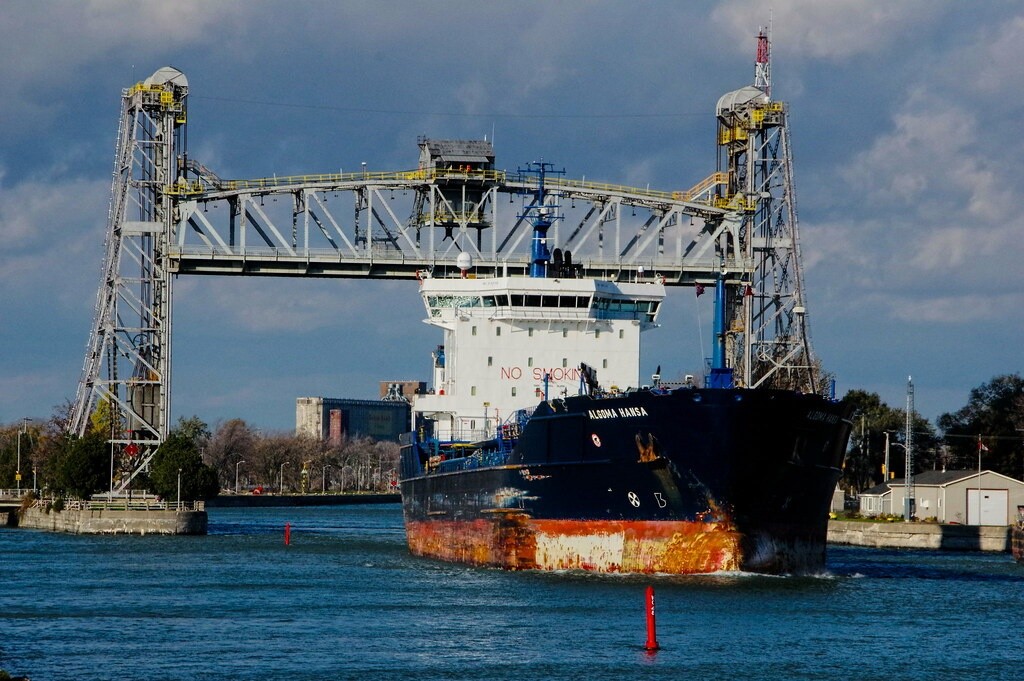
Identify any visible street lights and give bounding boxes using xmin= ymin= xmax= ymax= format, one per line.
xmin=891 ymin=443 xmax=910 ymax=522
xmin=281 ymin=462 xmax=289 ymax=493
xmin=341 ymin=466 xmax=349 ymax=492
xmin=18 ymin=431 xmax=22 ymax=488
xmin=322 ymin=465 xmax=331 ymax=493
xmin=236 ymin=461 xmax=245 ymax=493
xmin=884 ymin=432 xmax=889 ymax=482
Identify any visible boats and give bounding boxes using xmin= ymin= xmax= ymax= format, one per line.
xmin=398 ymin=162 xmax=856 ymax=589
